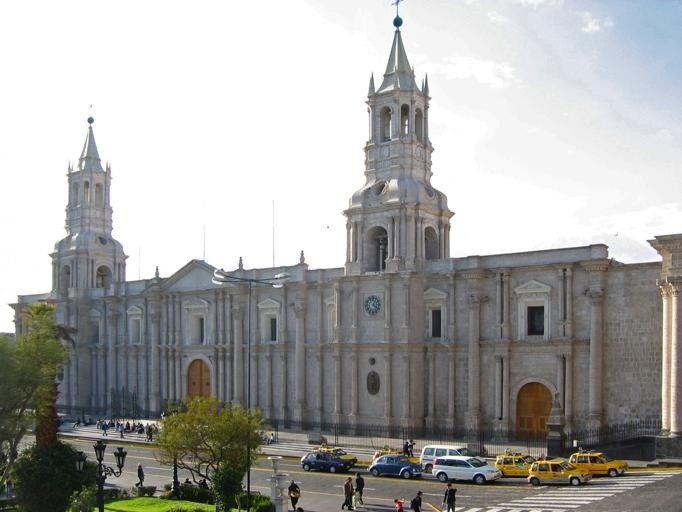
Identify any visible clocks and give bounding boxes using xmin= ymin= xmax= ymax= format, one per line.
xmin=364 ymin=295 xmax=382 ymax=316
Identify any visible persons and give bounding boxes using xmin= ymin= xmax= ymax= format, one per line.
xmin=407 ymin=439 xmax=416 ymax=456
xmin=197 ymin=479 xmax=203 ymax=487
xmin=265 ymin=433 xmax=275 ymax=445
xmin=403 ymin=441 xmax=411 ymax=457
xmin=344 ymin=477 xmax=355 ymax=508
xmin=288 ymin=478 xmax=300 ymax=511
xmin=93 ymin=417 xmax=158 ymax=443
xmin=354 ymin=471 xmax=365 ymax=505
xmin=297 ymin=506 xmax=304 ymax=511
xmin=410 ymin=490 xmax=423 ymax=512
xmin=393 ymin=497 xmax=405 ymax=511
xmin=184 ymin=478 xmax=192 ymax=484
xmin=202 ymin=478 xmax=209 ymax=489
xmin=442 ymin=483 xmax=457 ymax=512
xmin=341 ymin=477 xmax=354 ymax=511
xmin=73 ymin=416 xmax=81 ymax=428
xmin=135 ymin=463 xmax=145 ymax=488
xmin=352 ymin=487 xmax=362 ymax=510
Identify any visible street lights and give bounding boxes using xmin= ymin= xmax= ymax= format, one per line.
xmin=74 ymin=440 xmax=127 ymax=511
xmin=211 ymin=267 xmax=290 ymax=511
